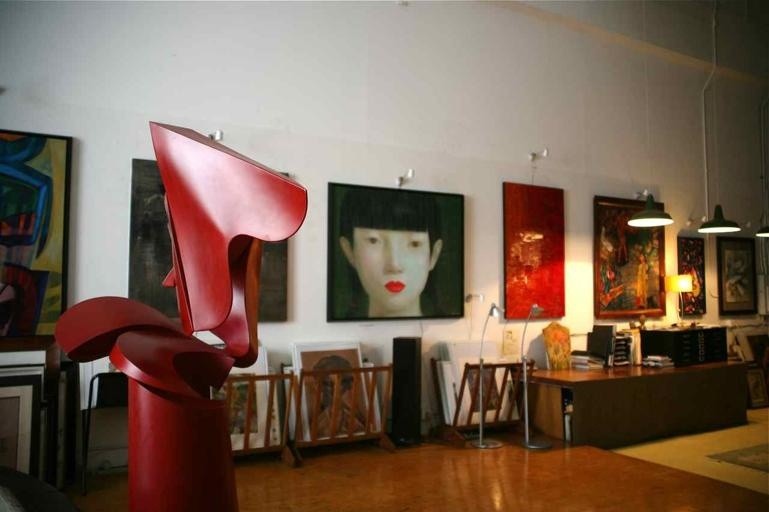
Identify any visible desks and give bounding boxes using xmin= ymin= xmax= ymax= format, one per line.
xmin=521 ymin=358 xmax=750 ymax=452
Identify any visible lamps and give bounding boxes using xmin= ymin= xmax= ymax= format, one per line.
xmin=754 ymin=114 xmax=769 ymax=239
xmin=520 ymin=303 xmax=552 ymax=451
xmin=697 ymin=115 xmax=741 ymax=235
xmin=670 ymin=272 xmax=693 ymax=324
xmin=469 ymin=302 xmax=506 ymax=450
xmin=627 ymin=188 xmax=674 ymax=228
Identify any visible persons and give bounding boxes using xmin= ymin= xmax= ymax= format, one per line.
xmin=339 ymin=188 xmax=443 ymax=318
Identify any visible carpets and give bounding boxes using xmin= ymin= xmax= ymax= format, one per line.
xmin=703 ymin=443 xmax=769 ymax=471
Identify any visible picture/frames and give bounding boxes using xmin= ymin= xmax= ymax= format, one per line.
xmin=324 ymin=180 xmax=467 ymax=325
xmin=0 ymin=129 xmax=75 ymax=353
xmin=677 ymin=235 xmax=706 ymax=316
xmin=591 ymin=195 xmax=668 ymax=317
xmin=289 ymin=337 xmax=371 ymax=440
xmin=0 ymin=371 xmax=44 ymax=479
xmin=716 ymin=235 xmax=758 ymax=316
